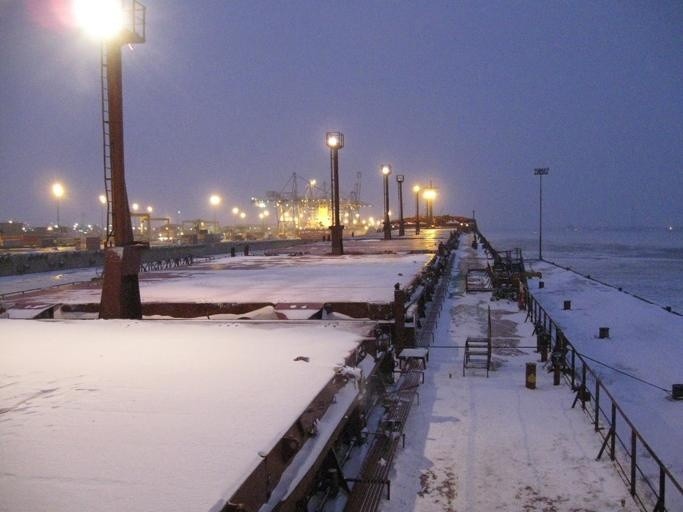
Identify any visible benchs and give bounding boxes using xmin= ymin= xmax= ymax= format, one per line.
xmin=342 ymin=361 xmax=424 ymax=512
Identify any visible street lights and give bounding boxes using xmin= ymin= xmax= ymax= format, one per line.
xmin=92 ymin=0 xmax=145 ymax=243
xmin=98 ymin=193 xmax=152 ymax=244
xmin=382 ymin=163 xmax=437 ymax=223
xmin=210 ymin=195 xmax=245 ymax=236
xmin=326 ymin=132 xmax=344 ymax=225
xmin=53 ymin=182 xmax=64 ymax=229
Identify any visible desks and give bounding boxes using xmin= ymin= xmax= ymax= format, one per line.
xmin=396 ymin=346 xmax=429 ymax=373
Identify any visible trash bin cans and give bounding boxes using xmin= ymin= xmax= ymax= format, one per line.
xmin=472 ymin=240 xmax=477 ymax=250
